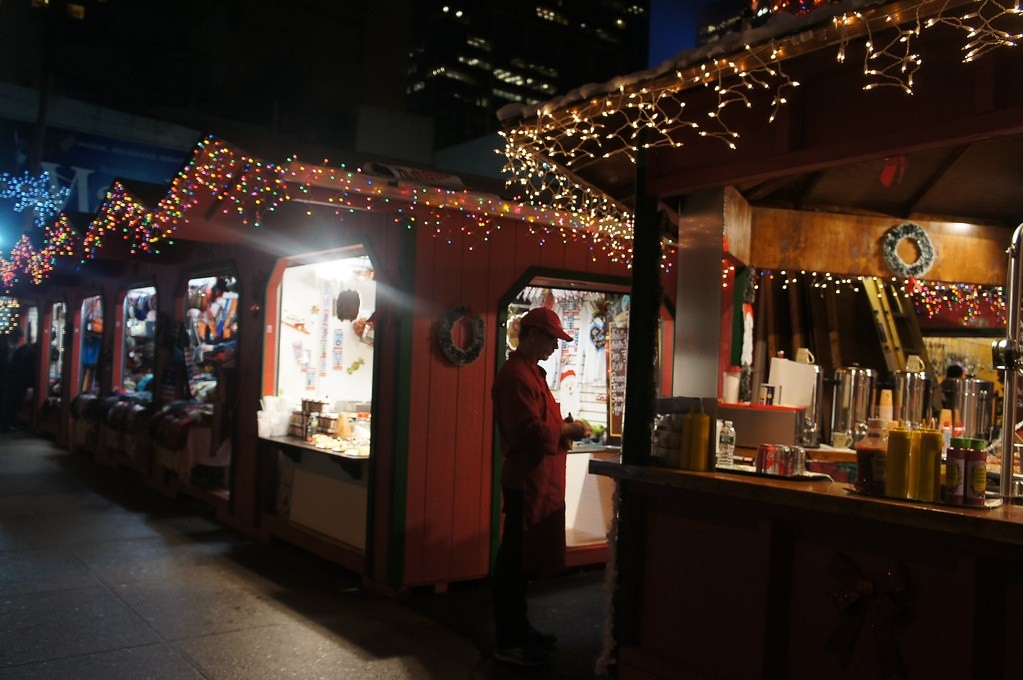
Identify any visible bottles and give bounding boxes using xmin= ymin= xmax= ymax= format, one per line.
xmin=853 ymin=389 xmax=986 ymax=507
xmin=680 ymin=398 xmax=696 ymax=470
xmin=717 ymin=421 xmax=736 ymax=469
xmin=689 ymin=397 xmax=710 ymax=471
xmin=335 ymin=411 xmax=371 ymax=444
xmin=306 ymin=412 xmax=318 ymax=442
xmin=723 ymin=366 xmax=741 ymax=404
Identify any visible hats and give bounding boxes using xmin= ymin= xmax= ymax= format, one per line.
xmin=520 ymin=307 xmax=573 ymax=342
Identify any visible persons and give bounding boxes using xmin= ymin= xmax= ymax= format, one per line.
xmin=491 ymin=308 xmax=591 ymax=649
xmin=0 ymin=327 xmax=58 ymax=418
xmin=941 ymin=365 xmax=964 ymax=405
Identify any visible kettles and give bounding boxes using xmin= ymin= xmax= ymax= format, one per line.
xmin=795 ymin=366 xmax=822 ymax=447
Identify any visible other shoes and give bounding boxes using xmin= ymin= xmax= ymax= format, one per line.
xmin=494 ymin=643 xmax=552 ymax=667
xmin=4 ymin=425 xmax=19 ymax=432
xmin=527 ymin=625 xmax=558 ymax=648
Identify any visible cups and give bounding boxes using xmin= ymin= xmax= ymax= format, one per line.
xmin=906 ymin=355 xmax=925 ymax=371
xmin=796 ymin=347 xmax=815 ymax=365
xmin=832 ymin=432 xmax=853 ymax=448
